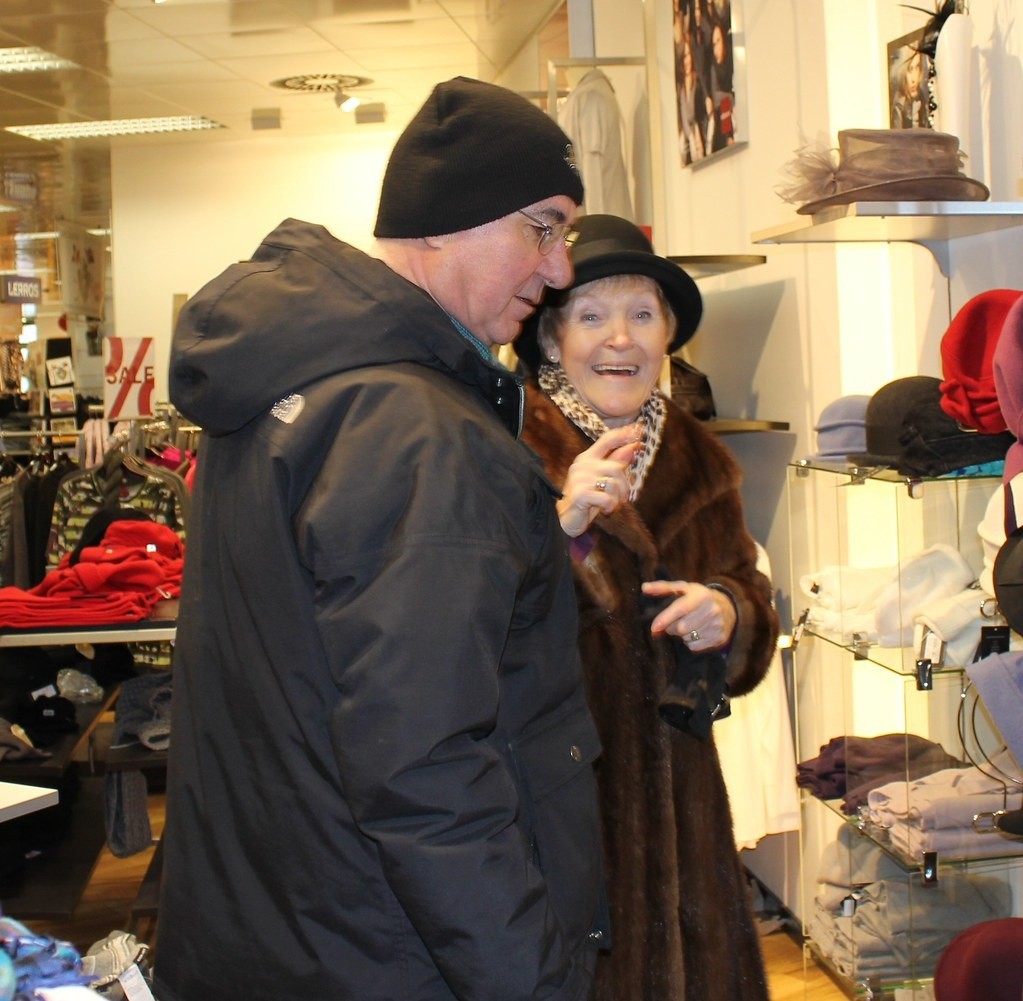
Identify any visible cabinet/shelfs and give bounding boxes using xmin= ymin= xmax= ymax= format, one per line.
xmin=0 ymin=615 xmax=175 ymax=1001
xmin=643 ymin=195 xmax=1023 ymax=1001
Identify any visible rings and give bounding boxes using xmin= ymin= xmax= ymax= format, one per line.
xmin=595 ymin=477 xmax=608 ymax=492
xmin=690 ymin=630 xmax=699 ymax=641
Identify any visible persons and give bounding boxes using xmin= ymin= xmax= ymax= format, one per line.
xmin=673 ymin=0 xmax=737 ymax=168
xmin=152 ymin=74 xmax=607 ymax=1001
xmin=510 ymin=214 xmax=781 ymax=1001
xmin=890 ymin=41 xmax=929 ymax=128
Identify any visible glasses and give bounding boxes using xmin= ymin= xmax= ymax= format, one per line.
xmin=517 ymin=209 xmax=581 ymax=256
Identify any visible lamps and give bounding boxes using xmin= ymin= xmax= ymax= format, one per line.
xmin=335 ymin=86 xmax=360 ymax=113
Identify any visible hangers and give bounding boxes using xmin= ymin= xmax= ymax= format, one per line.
xmin=0 ymin=407 xmax=203 ymax=493
xmin=570 ymin=63 xmax=616 ymax=92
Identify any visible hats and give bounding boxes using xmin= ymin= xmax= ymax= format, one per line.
xmin=372 ymin=77 xmax=583 ymax=238
xmin=933 ymin=916 xmax=1023 ymax=1001
xmin=993 ymin=526 xmax=1023 ymax=637
xmin=512 ymin=215 xmax=702 ymax=373
xmin=964 ymin=651 xmax=1023 ymax=768
xmin=814 ymin=291 xmax=1019 ymax=477
